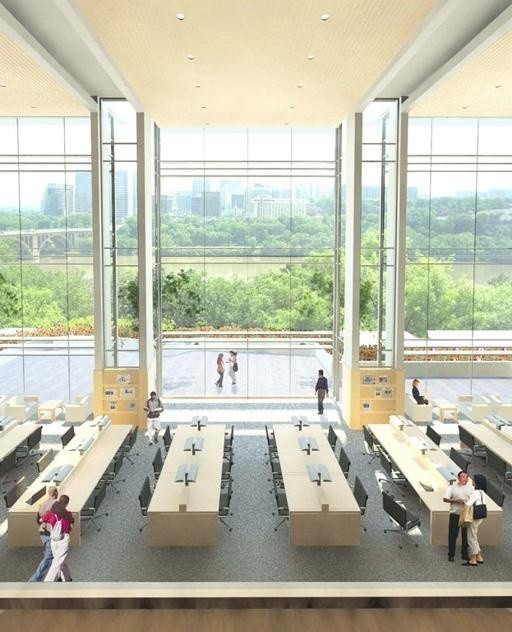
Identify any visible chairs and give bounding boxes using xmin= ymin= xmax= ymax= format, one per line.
xmin=361 ymin=424 xmax=512 ymax=552
xmin=264 ymin=424 xmax=368 ymax=553
xmin=137 ymin=424 xmax=234 ymax=550
xmin=0 ymin=424 xmax=144 ymax=551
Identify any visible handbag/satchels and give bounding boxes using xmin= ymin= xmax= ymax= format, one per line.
xmin=233 ymin=363 xmax=238 ymax=372
xmin=472 ymin=489 xmax=487 ymax=519
xmin=457 ymin=506 xmax=473 ymax=529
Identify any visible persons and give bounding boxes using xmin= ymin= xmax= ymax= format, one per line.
xmin=143 ymin=391 xmax=164 ymax=444
xmin=315 ymin=369 xmax=328 ymax=414
xmin=442 ymin=470 xmax=470 ymax=563
xmin=411 ymin=379 xmax=428 ymax=404
xmin=26 ymin=483 xmax=59 ymax=580
xmin=215 ymin=352 xmax=225 ymax=387
xmin=38 ymin=494 xmax=72 ymax=524
xmin=460 ymin=473 xmax=488 ymax=565
xmin=226 ymin=350 xmax=238 ymax=383
xmin=43 ymin=501 xmax=73 ymax=582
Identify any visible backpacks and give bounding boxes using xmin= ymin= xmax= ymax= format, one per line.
xmin=50 ymin=511 xmax=70 ymax=541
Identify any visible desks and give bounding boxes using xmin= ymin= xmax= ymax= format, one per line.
xmin=38 ymin=399 xmax=64 ymax=423
xmin=432 ymin=398 xmax=457 ymax=422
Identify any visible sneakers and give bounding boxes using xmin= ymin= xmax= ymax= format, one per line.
xmin=448 ymin=551 xmax=484 ymax=566
xmin=316 ymin=409 xmax=324 ymax=415
xmin=149 ymin=439 xmax=159 ymax=446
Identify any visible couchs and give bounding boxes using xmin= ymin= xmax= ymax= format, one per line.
xmin=404 ymin=391 xmax=432 ymax=423
xmin=457 ymin=393 xmax=511 ymax=424
xmin=64 ymin=394 xmax=89 ymax=423
xmin=1 ymin=395 xmax=40 ymax=423
